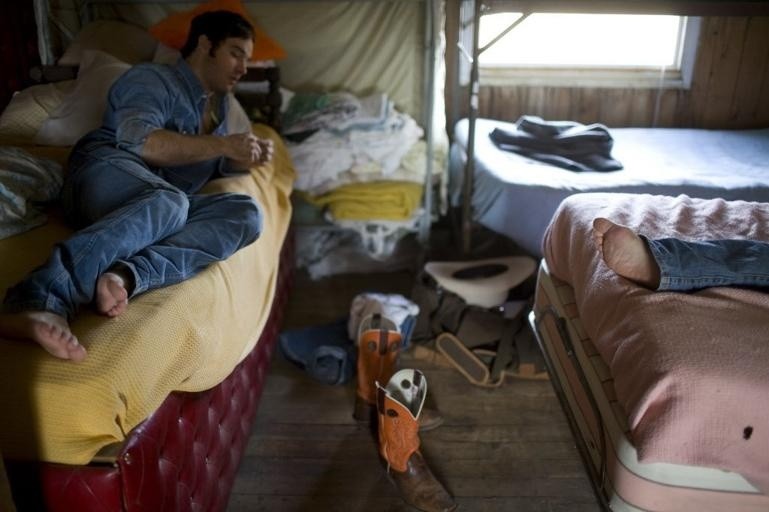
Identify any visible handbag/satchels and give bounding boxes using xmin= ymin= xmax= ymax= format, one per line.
xmin=410 ymin=272 xmax=532 ymax=371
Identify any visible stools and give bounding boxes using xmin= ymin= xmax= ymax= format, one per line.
xmin=415 ymin=249 xmax=543 ymax=351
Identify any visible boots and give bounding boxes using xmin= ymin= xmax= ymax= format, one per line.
xmin=374 ymin=368 xmax=459 ymax=512
xmin=353 ymin=312 xmax=443 ymax=433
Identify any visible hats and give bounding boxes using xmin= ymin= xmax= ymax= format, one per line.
xmin=424 ymin=256 xmax=538 ymax=309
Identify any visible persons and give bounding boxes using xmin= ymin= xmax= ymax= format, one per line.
xmin=2 ymin=8 xmax=277 ymax=362
xmin=589 ymin=215 xmax=769 ymax=294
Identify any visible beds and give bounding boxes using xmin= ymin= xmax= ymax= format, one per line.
xmin=451 ymin=102 xmax=768 ymax=244
xmin=2 ymin=54 xmax=439 ymax=298
xmin=2 ymin=42 xmax=282 ymax=511
xmin=530 ymin=191 xmax=768 ymax=511
